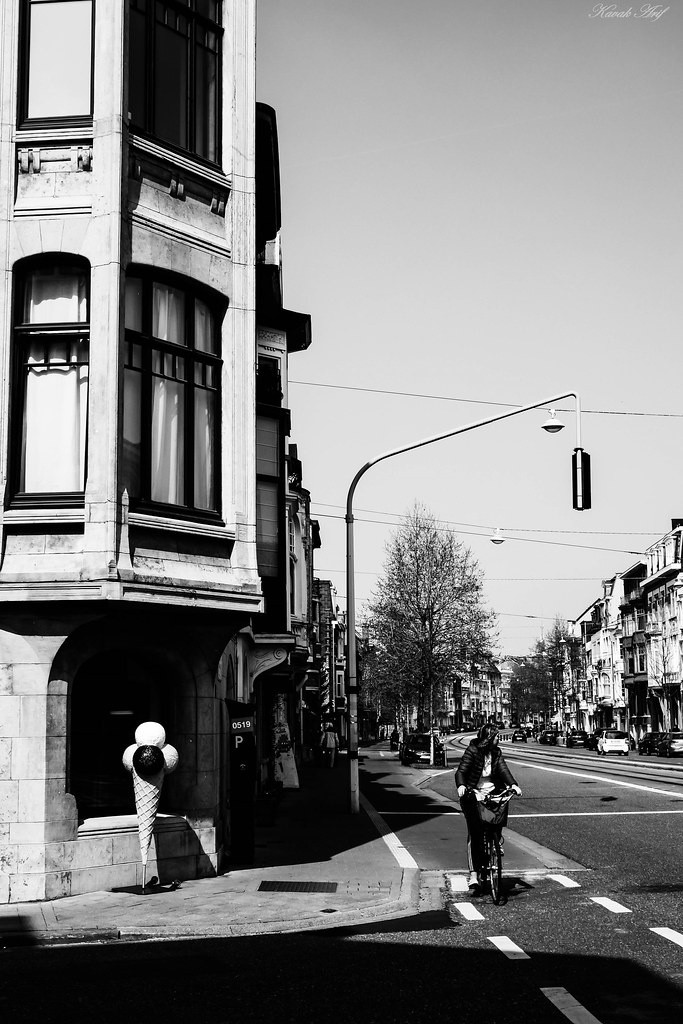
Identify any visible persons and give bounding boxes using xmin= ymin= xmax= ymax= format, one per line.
xmin=455 ymin=725 xmax=523 ymax=887
xmin=319 ymin=727 xmax=340 ymax=768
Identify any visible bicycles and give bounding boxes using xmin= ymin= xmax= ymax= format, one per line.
xmin=463 ymin=784 xmax=518 ymax=906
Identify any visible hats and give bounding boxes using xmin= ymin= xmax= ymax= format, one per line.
xmin=329 ymin=723 xmax=333 ymax=728
xmin=477 ymin=723 xmax=501 ymax=743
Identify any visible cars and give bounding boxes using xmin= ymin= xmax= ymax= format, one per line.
xmin=402 ymin=734 xmax=446 ymax=769
xmin=582 ymin=728 xmax=606 ymax=751
xmin=512 ymin=730 xmax=527 ymax=742
xmin=397 ymin=733 xmax=417 ymax=761
xmin=596 ymin=729 xmax=629 ymax=756
xmin=429 ymin=723 xmax=477 ymax=738
xmin=656 ymin=731 xmax=683 ymax=758
xmin=638 ymin=731 xmax=666 ymax=756
xmin=519 ymin=727 xmax=532 ymax=738
xmin=537 ymin=729 xmax=564 ymax=745
xmin=566 ymin=729 xmax=588 ymax=748
xmin=495 ymin=722 xmax=505 ymax=729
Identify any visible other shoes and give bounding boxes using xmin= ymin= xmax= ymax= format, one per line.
xmin=491 ymin=841 xmax=504 ymax=856
xmin=468 ymin=871 xmax=480 ymax=886
